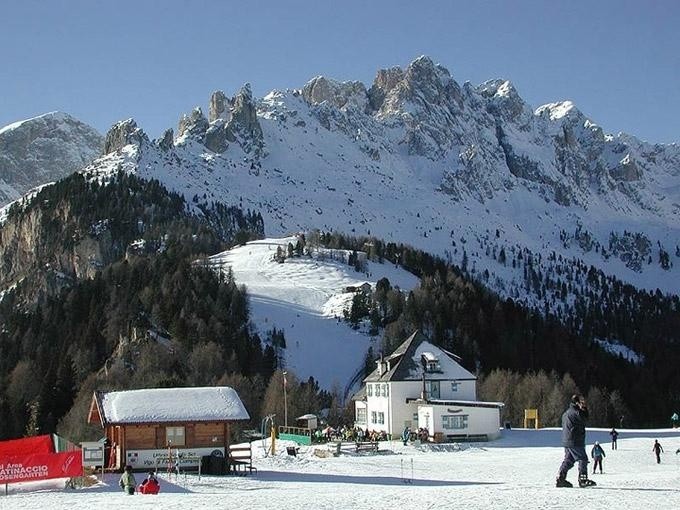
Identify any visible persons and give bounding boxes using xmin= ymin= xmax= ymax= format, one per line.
xmin=312 ymin=423 xmax=386 ymax=445
xmin=119 ymin=464 xmax=137 ymax=495
xmin=609 ymin=427 xmax=619 ymax=451
xmin=143 ymin=475 xmax=158 ymax=494
xmin=402 ymin=426 xmax=430 ymax=446
xmin=672 ymin=412 xmax=679 ymax=428
xmin=652 ymin=439 xmax=664 ymax=464
xmin=139 ymin=471 xmax=160 ymax=494
xmin=591 ymin=441 xmax=606 ymax=474
xmin=555 ymin=393 xmax=596 ymax=487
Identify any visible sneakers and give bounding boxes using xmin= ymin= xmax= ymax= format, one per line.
xmin=557 ymin=478 xmax=573 ymax=487
xmin=578 ymin=479 xmax=596 ymax=487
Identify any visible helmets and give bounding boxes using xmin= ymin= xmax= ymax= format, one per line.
xmin=572 ymin=394 xmax=584 ymax=404
